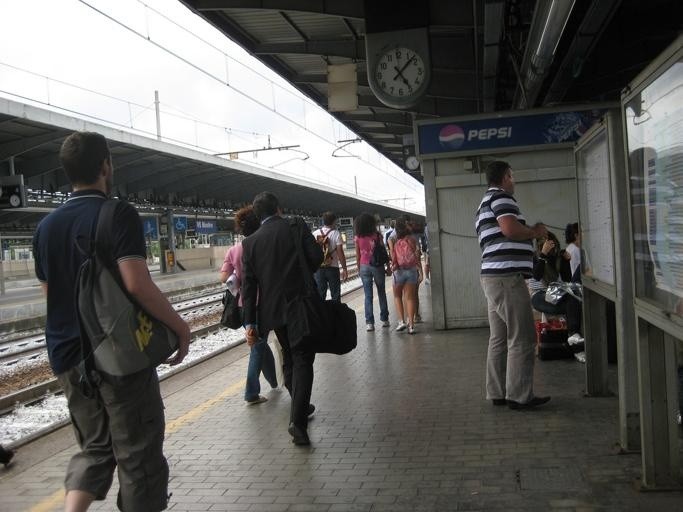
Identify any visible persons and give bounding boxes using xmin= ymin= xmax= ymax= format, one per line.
xmin=474 ymin=161 xmax=551 ymax=408
xmin=354 ymin=214 xmax=392 ymax=331
xmin=384 ymin=218 xmax=431 ymax=334
xmin=241 ymin=190 xmax=323 ymax=445
xmin=310 ymin=209 xmax=349 ymax=304
xmin=33 ymin=131 xmax=192 ymax=512
xmin=522 ymin=223 xmax=585 ymax=363
xmin=220 ymin=205 xmax=277 ymax=406
xmin=560 ymin=223 xmax=581 ymax=284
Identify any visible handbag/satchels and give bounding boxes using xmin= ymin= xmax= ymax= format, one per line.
xmin=318 ymin=299 xmax=356 ymax=356
xmin=219 ymin=289 xmax=242 ymax=329
xmin=369 ymin=231 xmax=388 ymax=266
xmin=544 ymin=282 xmax=582 ymax=304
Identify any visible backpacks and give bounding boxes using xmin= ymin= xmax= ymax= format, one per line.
xmin=73 ymin=200 xmax=179 ymax=385
xmin=392 ymin=236 xmax=417 ymax=267
xmin=316 ymin=229 xmax=337 ymax=267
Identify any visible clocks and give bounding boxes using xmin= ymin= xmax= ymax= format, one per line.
xmin=363 ymin=28 xmax=434 ymax=109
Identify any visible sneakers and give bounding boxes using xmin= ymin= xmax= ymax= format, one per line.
xmin=366 ymin=323 xmax=374 ymax=331
xmin=396 ymin=316 xmax=422 ymax=334
xmin=247 ymin=396 xmax=266 ymax=405
xmin=510 ymin=397 xmax=549 ymax=409
xmin=288 ymin=424 xmax=310 ymax=445
xmin=567 ymin=333 xmax=584 ymax=346
xmin=382 ymin=320 xmax=390 ymax=327
xmin=493 ymin=399 xmax=505 ymax=406
xmin=308 ymin=404 xmax=315 ymax=416
xmin=577 ymin=353 xmax=585 ymax=363
